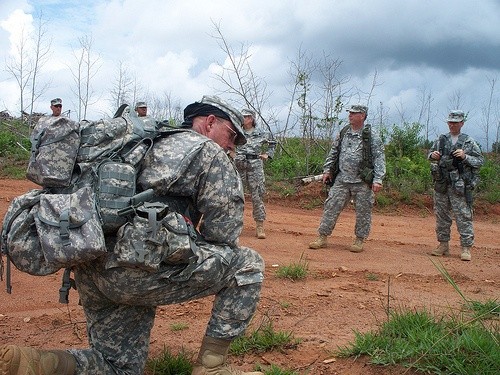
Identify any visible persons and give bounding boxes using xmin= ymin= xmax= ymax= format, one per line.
xmin=308 ymin=105 xmax=387 ymax=250
xmin=427 ymin=110 xmax=483 ymax=261
xmin=50 ymin=97 xmax=278 ymax=238
xmin=0 ymin=94 xmax=265 ymax=375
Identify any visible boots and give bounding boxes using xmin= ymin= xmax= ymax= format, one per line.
xmin=351 ymin=237 xmax=364 ymax=252
xmin=191 ymin=336 xmax=265 ymax=375
xmin=460 ymin=246 xmax=471 ymax=260
xmin=431 ymin=242 xmax=449 ymax=256
xmin=256 ymin=221 xmax=265 ymax=239
xmin=0 ymin=344 xmax=77 ymax=375
xmin=309 ymin=234 xmax=327 ymax=248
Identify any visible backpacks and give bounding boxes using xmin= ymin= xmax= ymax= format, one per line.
xmin=0 ymin=104 xmax=197 ymax=303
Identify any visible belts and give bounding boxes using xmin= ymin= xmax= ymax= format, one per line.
xmin=246 ymin=155 xmax=260 ymax=159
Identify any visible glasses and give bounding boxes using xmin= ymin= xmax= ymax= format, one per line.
xmin=448 ymin=120 xmax=463 ymax=123
xmin=54 ymin=105 xmax=62 ymax=108
xmin=216 ymin=117 xmax=238 ymax=143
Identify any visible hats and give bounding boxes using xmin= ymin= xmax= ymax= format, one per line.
xmin=241 ymin=109 xmax=256 ymax=119
xmin=201 ymin=94 xmax=247 ymax=145
xmin=446 ymin=111 xmax=465 ymax=122
xmin=51 ymin=98 xmax=62 ymax=106
xmin=136 ymin=102 xmax=147 ymax=107
xmin=346 ymin=105 xmax=369 ymax=116
